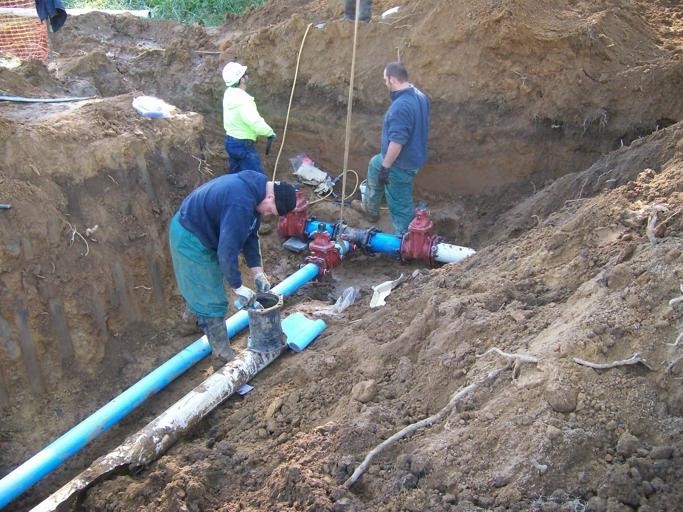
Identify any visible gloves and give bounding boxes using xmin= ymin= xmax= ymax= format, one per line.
xmin=255 ymin=273 xmax=270 ymax=292
xmin=377 ymin=167 xmax=391 ymax=185
xmin=234 ymin=284 xmax=255 ymax=306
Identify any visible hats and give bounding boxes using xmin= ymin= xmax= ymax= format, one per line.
xmin=274 ymin=180 xmax=297 ymax=216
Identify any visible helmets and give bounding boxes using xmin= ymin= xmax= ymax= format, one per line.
xmin=222 ymin=63 xmax=247 ymax=86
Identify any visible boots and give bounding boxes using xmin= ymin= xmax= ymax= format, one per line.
xmin=203 ymin=316 xmax=236 ymax=370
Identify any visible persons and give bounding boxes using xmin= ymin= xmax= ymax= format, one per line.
xmin=350 ymin=61 xmax=432 ymax=237
xmin=335 ymin=0 xmax=373 ymax=25
xmin=219 ymin=61 xmax=278 ymax=175
xmin=167 ymin=168 xmax=297 ymax=375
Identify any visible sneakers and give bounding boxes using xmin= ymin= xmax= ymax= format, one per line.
xmin=352 ymin=199 xmax=378 ymax=223
